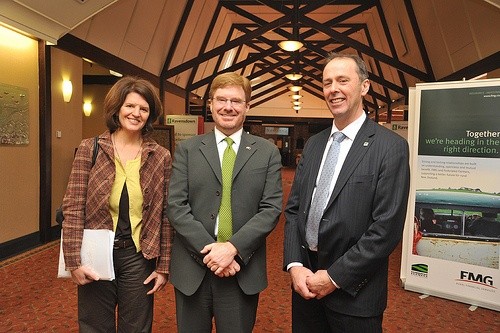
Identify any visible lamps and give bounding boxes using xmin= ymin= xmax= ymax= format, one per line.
xmin=289 ymin=94 xmax=303 ymax=100
xmin=291 ymin=106 xmax=302 ymax=110
xmin=285 ymin=73 xmax=303 ymax=80
xmin=287 ymin=86 xmax=303 ymax=92
xmin=278 ymin=40 xmax=304 ymax=51
xmin=290 ymin=101 xmax=303 ymax=105
xmin=64 ymin=81 xmax=72 ymax=102
xmin=83 ymin=109 xmax=91 ymax=116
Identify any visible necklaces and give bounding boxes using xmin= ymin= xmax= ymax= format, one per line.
xmin=112 ymin=132 xmax=143 ymax=160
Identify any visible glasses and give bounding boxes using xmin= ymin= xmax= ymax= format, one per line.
xmin=212 ymin=97 xmax=247 ymax=105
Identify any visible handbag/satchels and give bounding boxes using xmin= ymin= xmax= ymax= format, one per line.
xmin=56 ymin=134 xmax=99 ymax=225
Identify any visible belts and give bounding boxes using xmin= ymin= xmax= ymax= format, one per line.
xmin=113 ymin=238 xmax=134 ymax=249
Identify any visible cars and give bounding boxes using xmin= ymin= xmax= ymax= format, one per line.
xmin=411 ymin=185 xmax=500 ymax=270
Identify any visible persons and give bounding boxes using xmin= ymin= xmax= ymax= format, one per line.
xmin=172 ymin=71 xmax=283 ymax=333
xmin=61 ymin=77 xmax=172 ymax=333
xmin=283 ymin=56 xmax=409 ymax=333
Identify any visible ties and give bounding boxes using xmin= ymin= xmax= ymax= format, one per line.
xmin=217 ymin=137 xmax=237 ymax=242
xmin=305 ymin=132 xmax=346 ymax=250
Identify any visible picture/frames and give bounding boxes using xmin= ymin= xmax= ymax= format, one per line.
xmin=155 ymin=124 xmax=175 ymax=155
